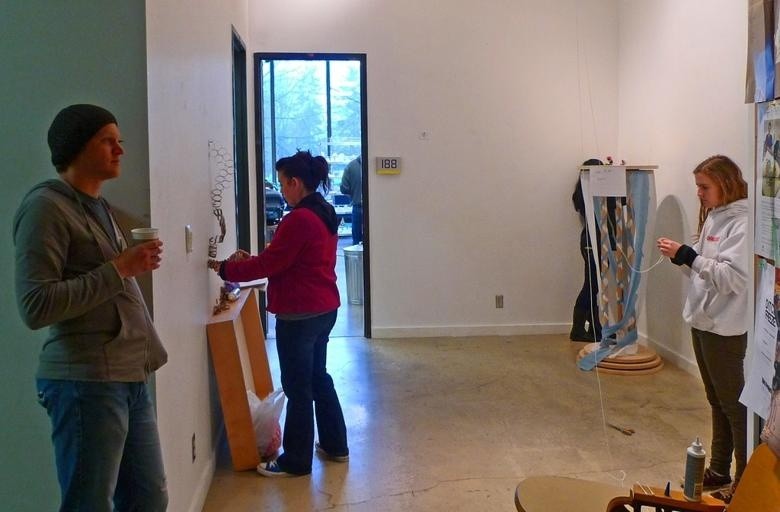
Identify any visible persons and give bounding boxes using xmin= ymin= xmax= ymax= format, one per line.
xmin=340 ymin=152 xmax=364 ymax=246
xmin=12 ymin=102 xmax=169 ymax=508
xmin=207 ymin=150 xmax=351 ymax=479
xmin=570 ymin=156 xmax=630 ymax=343
xmin=655 ymin=154 xmax=751 ymax=505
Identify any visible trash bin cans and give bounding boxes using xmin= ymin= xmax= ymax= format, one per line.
xmin=343 ymin=245 xmax=364 ymax=304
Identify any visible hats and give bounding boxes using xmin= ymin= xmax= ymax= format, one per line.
xmin=47 ymin=105 xmax=117 ymax=164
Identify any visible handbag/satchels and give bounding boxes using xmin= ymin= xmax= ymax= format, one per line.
xmin=242 ymin=388 xmax=286 ymax=456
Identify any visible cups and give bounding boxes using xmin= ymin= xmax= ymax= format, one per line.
xmin=132 ymin=227 xmax=160 ymax=248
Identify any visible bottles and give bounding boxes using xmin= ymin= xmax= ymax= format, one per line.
xmin=683 ymin=436 xmax=705 ymax=503
xmin=228 ymin=288 xmax=241 ymax=302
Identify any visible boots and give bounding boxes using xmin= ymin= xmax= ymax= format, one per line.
xmin=570 ymin=306 xmax=616 ymax=341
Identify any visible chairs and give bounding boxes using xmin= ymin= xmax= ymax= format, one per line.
xmin=515 ymin=442 xmax=780 ymax=512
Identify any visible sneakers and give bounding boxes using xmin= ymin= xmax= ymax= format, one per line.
xmin=256 ymin=460 xmax=311 ymax=477
xmin=680 ymin=467 xmax=736 ymax=504
xmin=315 ymin=440 xmax=349 ymax=462
